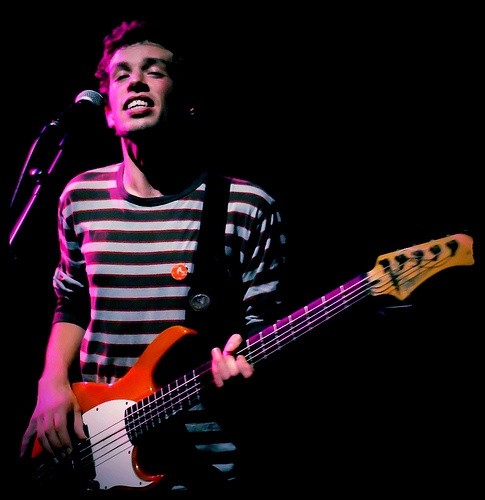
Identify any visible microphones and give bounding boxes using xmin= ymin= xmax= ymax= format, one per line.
xmin=51 ymin=89 xmax=103 ymax=128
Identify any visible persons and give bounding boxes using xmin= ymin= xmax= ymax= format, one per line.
xmin=20 ymin=18 xmax=286 ymax=500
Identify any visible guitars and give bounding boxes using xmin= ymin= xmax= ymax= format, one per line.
xmin=30 ymin=229 xmax=476 ymax=500
